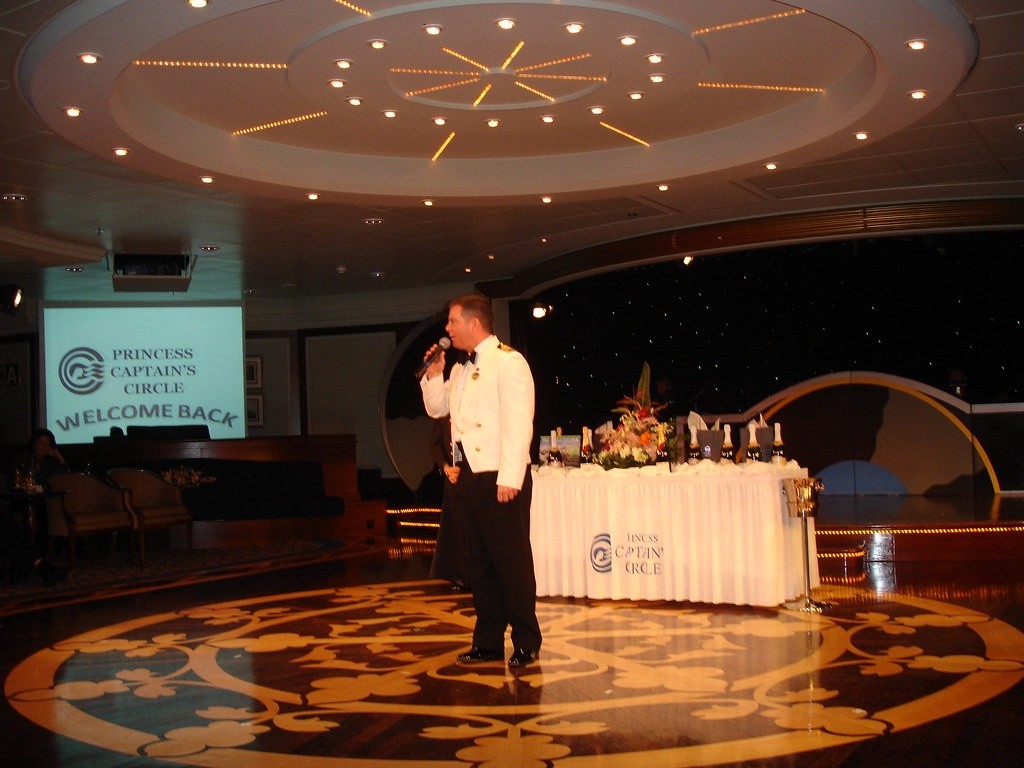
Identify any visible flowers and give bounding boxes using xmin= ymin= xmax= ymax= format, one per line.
xmin=581 ymin=389 xmax=676 ymax=469
xmin=157 ymin=461 xmax=218 ymax=490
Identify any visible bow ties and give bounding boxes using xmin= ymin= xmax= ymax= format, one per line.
xmin=458 ymin=350 xmax=476 ymax=365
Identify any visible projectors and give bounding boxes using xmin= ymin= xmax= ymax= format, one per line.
xmin=113 ymin=252 xmax=190 ymax=292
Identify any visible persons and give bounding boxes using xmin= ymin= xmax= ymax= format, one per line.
xmin=14 ymin=427 xmax=66 ymax=486
xmin=419 ymin=293 xmax=544 ymax=670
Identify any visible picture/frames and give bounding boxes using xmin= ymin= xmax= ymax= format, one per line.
xmin=246 ymin=356 xmax=264 ymax=391
xmin=246 ymin=393 xmax=264 ymax=428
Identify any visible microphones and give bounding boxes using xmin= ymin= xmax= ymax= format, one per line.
xmin=415 ymin=336 xmax=451 ymax=378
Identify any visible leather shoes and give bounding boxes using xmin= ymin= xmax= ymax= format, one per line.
xmin=456 ymin=646 xmax=504 ymax=663
xmin=508 ymin=647 xmax=540 ymax=666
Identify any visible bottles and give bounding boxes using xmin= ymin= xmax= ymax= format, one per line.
xmin=688 ymin=425 xmax=702 ymax=464
xmin=746 ymin=424 xmax=762 ymax=464
xmin=771 ymin=422 xmax=787 ymax=465
xmin=720 ymin=424 xmax=734 ymax=465
xmin=547 ymin=430 xmax=563 ymax=467
xmin=579 ymin=427 xmax=594 ymax=470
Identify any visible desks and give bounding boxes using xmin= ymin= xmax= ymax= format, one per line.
xmin=528 ymin=465 xmax=823 ymax=609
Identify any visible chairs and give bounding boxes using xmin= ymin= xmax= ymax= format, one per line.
xmin=42 ymin=471 xmax=139 ymax=565
xmin=105 ymin=466 xmax=194 ymax=562
